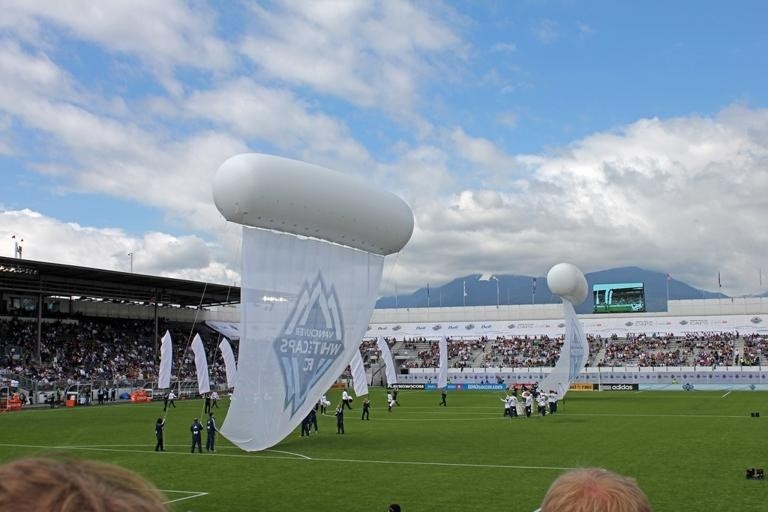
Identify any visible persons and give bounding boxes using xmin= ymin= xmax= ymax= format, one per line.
xmin=334 ymin=403 xmax=345 ymax=434
xmin=361 ymin=396 xmax=370 ymax=420
xmin=163 ymin=392 xmax=169 ymax=413
xmin=438 ymin=390 xmax=448 ymax=406
xmin=446 ymin=334 xmax=565 ymax=367
xmin=154 ymin=418 xmax=166 ymax=450
xmin=342 ymin=388 xmax=352 ymax=409
xmin=319 ymin=394 xmax=329 ymax=414
xmin=346 ymin=376 xmax=350 ymax=387
xmin=386 ymin=390 xmax=395 ymax=412
xmin=205 ymin=412 xmax=219 ymax=452
xmin=392 ymin=387 xmax=401 ymax=406
xmin=189 ymin=418 xmax=205 ymax=453
xmin=539 ymin=465 xmax=651 ymax=511
xmin=2 ymin=453 xmax=172 ymax=512
xmin=204 ymin=394 xmax=211 ymax=414
xmin=358 ymin=336 xmax=441 ymax=367
xmin=498 ymin=381 xmax=559 ymax=417
xmin=27 ymin=386 xmax=34 ymax=405
xmin=386 ymin=503 xmax=401 ymax=511
xmin=0 ymin=317 xmax=238 ymax=408
xmin=586 ymin=329 xmax=767 ymax=367
xmin=301 ymin=418 xmax=310 ymax=437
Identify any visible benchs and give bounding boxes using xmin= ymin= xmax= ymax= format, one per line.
xmin=376 ymin=336 xmax=764 ymax=367
